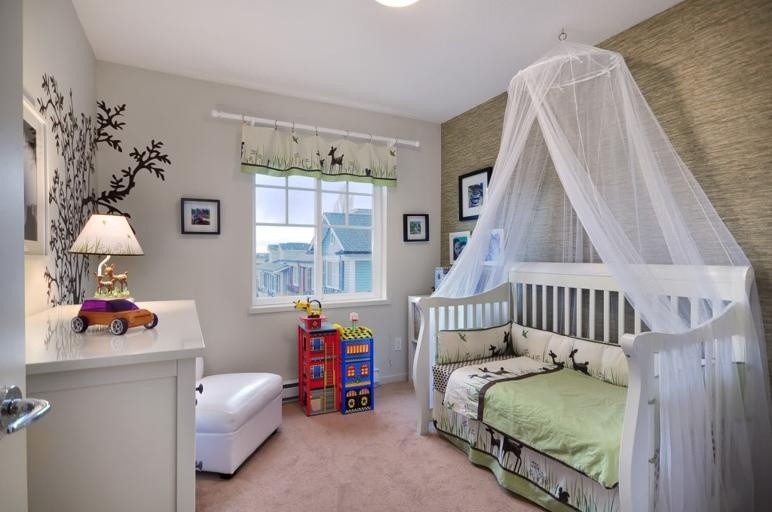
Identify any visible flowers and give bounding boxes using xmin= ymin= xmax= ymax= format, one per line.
xmin=294 ymin=297 xmax=325 ymax=317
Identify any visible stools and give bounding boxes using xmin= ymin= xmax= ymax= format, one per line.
xmin=193 ymin=370 xmax=285 ymax=481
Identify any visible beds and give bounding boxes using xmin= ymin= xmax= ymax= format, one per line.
xmin=412 ymin=262 xmax=756 ymax=512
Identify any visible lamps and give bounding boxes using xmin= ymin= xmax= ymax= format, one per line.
xmin=65 ymin=214 xmax=146 ymax=302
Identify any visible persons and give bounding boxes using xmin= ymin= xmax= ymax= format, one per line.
xmin=469 ymin=189 xmax=481 ymax=206
xmin=453 ymin=239 xmax=465 ymax=260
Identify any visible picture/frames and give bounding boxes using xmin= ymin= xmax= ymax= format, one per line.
xmin=458 ymin=166 xmax=492 ymax=221
xmin=403 ymin=213 xmax=429 ymax=242
xmin=180 ymin=197 xmax=221 ymax=235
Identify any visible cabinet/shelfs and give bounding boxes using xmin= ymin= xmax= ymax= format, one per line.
xmin=28 ymin=298 xmax=208 ymax=511
xmin=298 ymin=321 xmax=375 ymax=418
